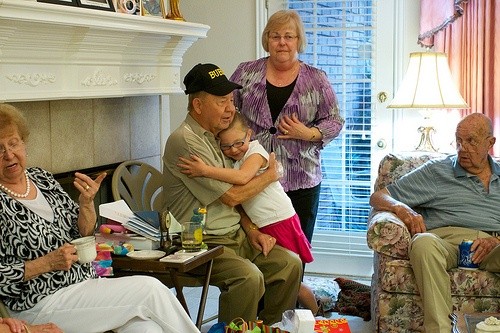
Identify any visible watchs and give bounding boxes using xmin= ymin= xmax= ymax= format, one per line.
xmin=246 ymin=225 xmax=258 ymax=234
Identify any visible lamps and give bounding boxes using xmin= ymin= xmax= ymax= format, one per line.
xmin=385 ymin=47 xmax=472 ymax=158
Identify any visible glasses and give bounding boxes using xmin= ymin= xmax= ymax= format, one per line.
xmin=0 ymin=137 xmax=24 ymax=159
xmin=269 ymin=35 xmax=300 ymax=43
xmin=450 ymin=135 xmax=490 ymax=149
xmin=220 ymin=130 xmax=248 ymax=151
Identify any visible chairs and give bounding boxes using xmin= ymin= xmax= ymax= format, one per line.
xmin=111 ymin=161 xmax=220 ymax=331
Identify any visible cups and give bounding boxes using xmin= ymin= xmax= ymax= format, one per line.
xmin=458 ymin=243 xmax=482 ymax=270
xmin=181 ymin=222 xmax=202 ymax=252
xmin=70 ymin=236 xmax=97 ymax=265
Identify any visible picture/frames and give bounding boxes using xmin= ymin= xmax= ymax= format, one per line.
xmin=37 ymin=0 xmax=167 ymax=19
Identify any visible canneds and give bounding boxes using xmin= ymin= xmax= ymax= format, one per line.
xmin=459 ymin=239 xmax=479 ymax=270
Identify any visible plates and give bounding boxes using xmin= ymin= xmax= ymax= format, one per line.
xmin=158 ymin=254 xmax=194 ymax=263
xmin=174 ymin=249 xmax=208 ymax=256
xmin=126 ymin=250 xmax=166 ymax=260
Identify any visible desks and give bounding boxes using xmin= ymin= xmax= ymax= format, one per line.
xmin=109 ymin=243 xmax=224 ymax=330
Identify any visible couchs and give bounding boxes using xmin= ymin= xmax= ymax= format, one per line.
xmin=366 ymin=153 xmax=500 ymax=333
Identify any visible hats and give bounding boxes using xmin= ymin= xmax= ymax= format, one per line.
xmin=183 ymin=63 xmax=243 ymax=96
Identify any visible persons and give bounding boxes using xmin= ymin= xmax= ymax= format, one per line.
xmin=0 ymin=104 xmax=201 ymax=333
xmin=228 ymin=10 xmax=345 ymax=281
xmin=177 ymin=110 xmax=319 ymax=314
xmin=163 ymin=63 xmax=304 ymax=326
xmin=370 ymin=113 xmax=500 ymax=333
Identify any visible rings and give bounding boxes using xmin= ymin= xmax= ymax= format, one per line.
xmin=85 ymin=185 xmax=89 ymax=190
xmin=284 ymin=130 xmax=288 ymax=135
xmin=269 ymin=238 xmax=272 ymax=240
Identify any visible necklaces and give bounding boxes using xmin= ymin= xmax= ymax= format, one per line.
xmin=278 ymin=66 xmax=296 ymax=86
xmin=0 ymin=171 xmax=30 ymax=198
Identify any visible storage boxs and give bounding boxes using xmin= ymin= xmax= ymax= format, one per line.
xmin=294 ymin=309 xmax=316 ymax=333
xmin=94 ymin=233 xmax=161 ymax=250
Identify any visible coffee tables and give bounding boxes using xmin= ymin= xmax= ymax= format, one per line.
xmin=448 ymin=310 xmax=500 ymax=333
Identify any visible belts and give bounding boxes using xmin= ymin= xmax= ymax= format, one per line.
xmin=486 ymin=231 xmax=499 ymax=237
